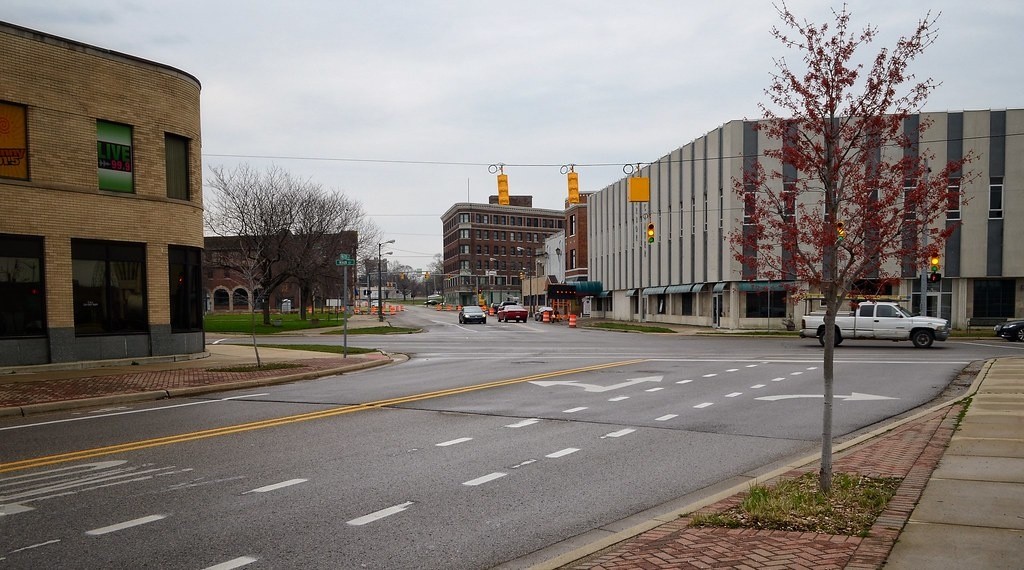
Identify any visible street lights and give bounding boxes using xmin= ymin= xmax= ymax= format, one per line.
xmin=490 ymin=258 xmax=503 ymax=303
xmin=378 ymin=239 xmax=396 ymax=321
xmin=517 ymin=247 xmax=532 ymax=312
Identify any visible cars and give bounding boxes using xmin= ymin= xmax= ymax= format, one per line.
xmin=993 ymin=321 xmax=1024 ymax=342
xmin=424 ymin=300 xmax=439 ymax=306
xmin=458 ymin=306 xmax=486 ymax=324
xmin=534 ymin=307 xmax=555 ymax=321
xmin=491 ymin=301 xmax=528 ymax=323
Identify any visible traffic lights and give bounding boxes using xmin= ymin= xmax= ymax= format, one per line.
xmin=646 ymin=222 xmax=656 ymax=244
xmin=424 ymin=274 xmax=429 ymax=280
xmin=930 ymin=251 xmax=939 ymax=271
xmin=837 ymin=222 xmax=845 ymax=241
xmin=519 ymin=272 xmax=524 ymax=280
xmin=930 ymin=273 xmax=941 ymax=283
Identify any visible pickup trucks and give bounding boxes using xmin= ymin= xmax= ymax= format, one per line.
xmin=799 ymin=301 xmax=949 ymax=349
